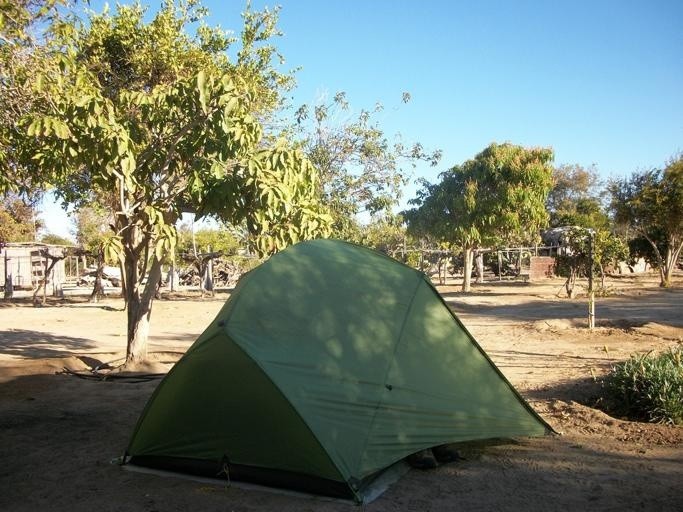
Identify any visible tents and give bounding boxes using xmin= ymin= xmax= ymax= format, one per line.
xmin=119 ymin=238 xmax=561 ymax=507
